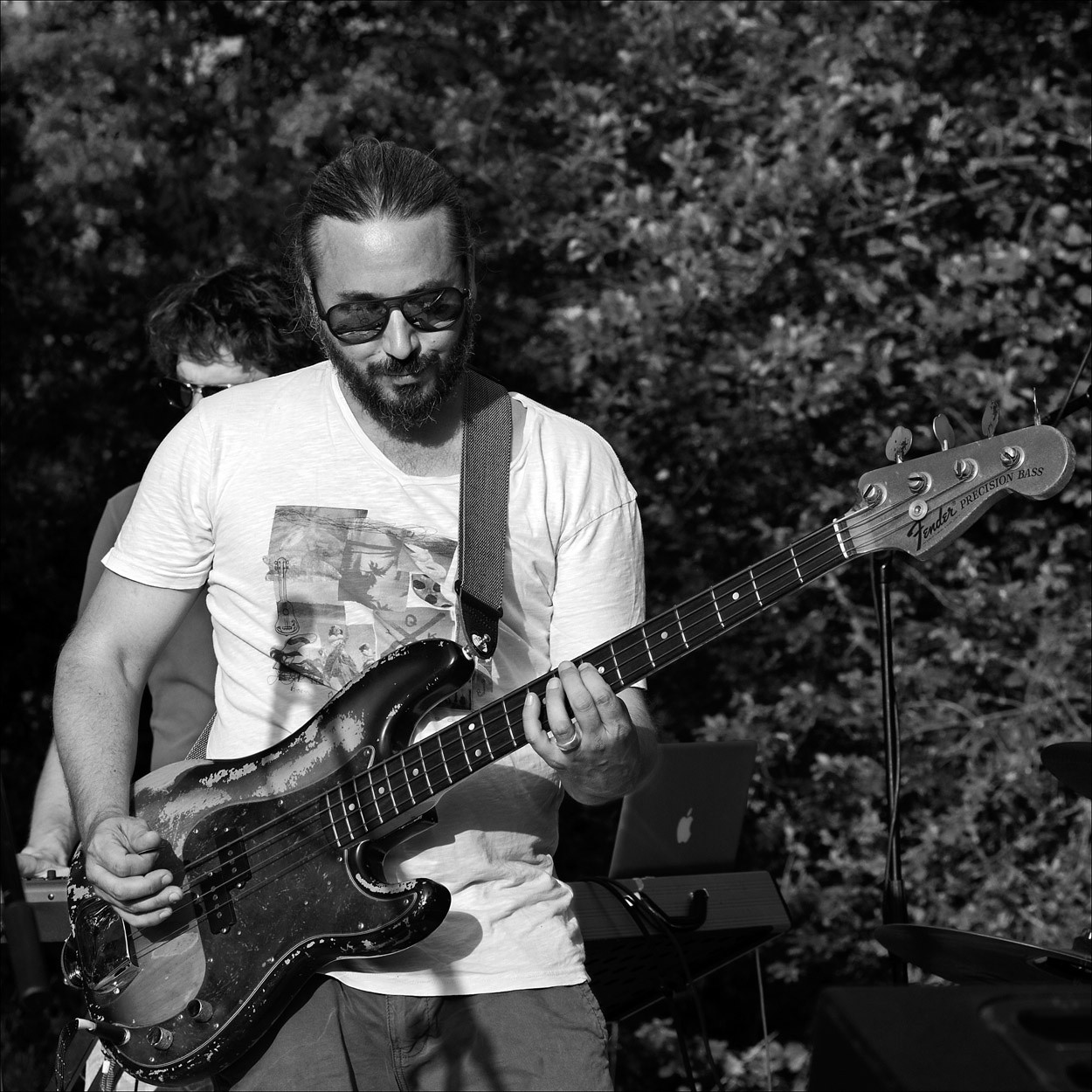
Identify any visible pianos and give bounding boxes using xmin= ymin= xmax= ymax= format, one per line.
xmin=20 ymin=867 xmax=794 ymax=1092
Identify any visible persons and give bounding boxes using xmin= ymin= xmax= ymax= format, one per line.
xmin=52 ymin=139 xmax=655 ymax=1092
xmin=14 ymin=267 xmax=311 ymax=1092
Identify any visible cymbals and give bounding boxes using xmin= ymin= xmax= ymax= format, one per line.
xmin=1041 ymin=741 xmax=1092 ymax=800
xmin=874 ymin=924 xmax=1092 ymax=986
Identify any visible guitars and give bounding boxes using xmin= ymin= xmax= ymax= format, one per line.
xmin=66 ymin=387 xmax=1075 ymax=1087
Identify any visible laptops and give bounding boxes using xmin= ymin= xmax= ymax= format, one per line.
xmin=608 ymin=739 xmax=756 ymax=879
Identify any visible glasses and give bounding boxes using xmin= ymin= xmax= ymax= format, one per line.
xmin=308 ymin=253 xmax=470 ymax=344
xmin=158 ymin=375 xmax=234 ymax=411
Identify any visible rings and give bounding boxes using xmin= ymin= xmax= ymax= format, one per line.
xmin=555 ymin=731 xmax=580 ymax=752
